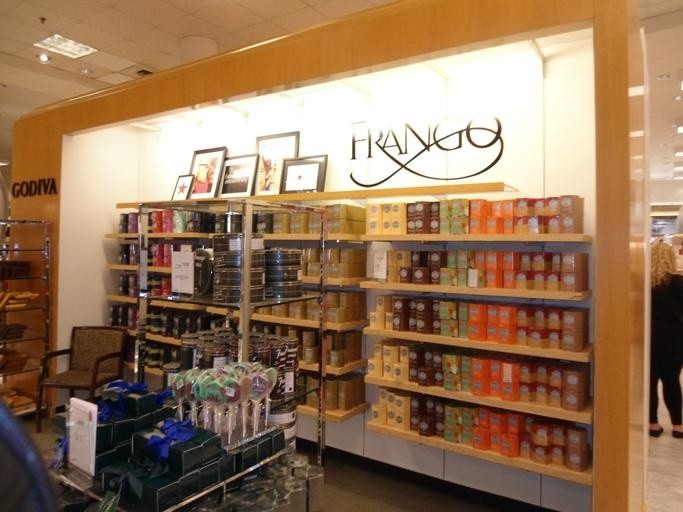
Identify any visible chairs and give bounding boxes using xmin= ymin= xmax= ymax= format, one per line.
xmin=36 ymin=327 xmax=128 ymax=432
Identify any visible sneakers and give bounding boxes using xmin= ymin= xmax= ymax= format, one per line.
xmin=672 ymin=430 xmax=683 ymax=439
xmin=650 ymin=428 xmax=663 ymax=438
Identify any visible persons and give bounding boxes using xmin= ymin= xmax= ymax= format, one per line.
xmin=650 ymin=240 xmax=683 ymax=440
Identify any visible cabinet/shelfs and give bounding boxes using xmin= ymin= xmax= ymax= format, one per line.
xmin=206 ymin=229 xmax=362 ymax=420
xmin=361 ymin=234 xmax=595 ymax=486
xmin=52 ymin=441 xmax=293 ymax=512
xmin=135 ymin=201 xmax=323 ymax=512
xmin=2 ymin=218 xmax=49 ymax=421
xmin=105 ymin=233 xmax=205 ymax=375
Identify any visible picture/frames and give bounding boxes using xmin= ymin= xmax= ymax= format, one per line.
xmin=170 ymin=175 xmax=192 ymax=199
xmin=279 ymin=154 xmax=327 ymax=192
xmin=190 ymin=146 xmax=227 ymax=199
xmin=255 ymin=131 xmax=300 ymax=194
xmin=220 ymin=153 xmax=259 ymax=197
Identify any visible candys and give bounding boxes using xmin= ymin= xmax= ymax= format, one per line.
xmin=171 ymin=361 xmax=277 ymax=444
